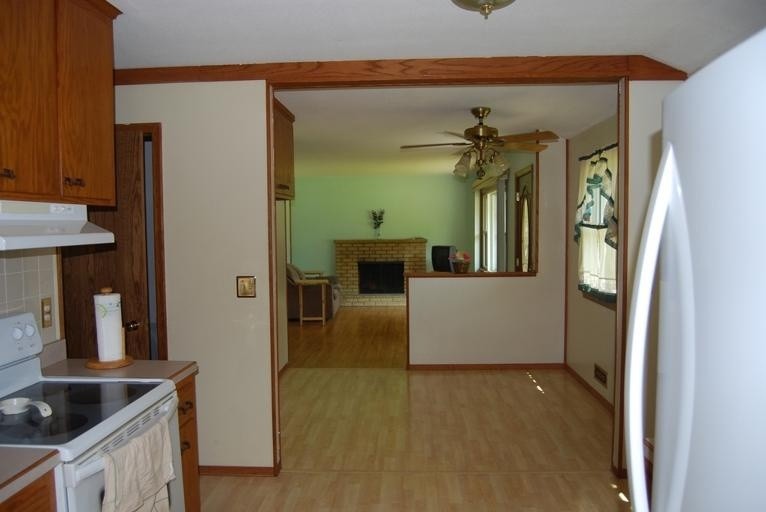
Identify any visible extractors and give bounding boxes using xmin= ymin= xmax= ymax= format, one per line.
xmin=0 ymin=200 xmax=116 ymax=252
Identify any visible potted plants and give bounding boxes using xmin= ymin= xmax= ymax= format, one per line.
xmin=370 ymin=208 xmax=385 ymax=239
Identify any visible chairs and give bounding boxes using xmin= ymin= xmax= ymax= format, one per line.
xmin=286 ymin=263 xmax=341 ymax=322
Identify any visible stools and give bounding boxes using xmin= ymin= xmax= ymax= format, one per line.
xmin=295 ymin=278 xmax=330 ymax=327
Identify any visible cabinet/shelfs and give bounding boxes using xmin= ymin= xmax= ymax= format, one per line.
xmin=174 ymin=372 xmax=201 ymax=512
xmin=0 ymin=468 xmax=58 ymax=512
xmin=274 ymin=95 xmax=295 ymax=200
xmin=0 ymin=0 xmax=118 ymax=207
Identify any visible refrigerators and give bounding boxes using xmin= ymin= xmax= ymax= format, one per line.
xmin=620 ymin=24 xmax=766 ymax=512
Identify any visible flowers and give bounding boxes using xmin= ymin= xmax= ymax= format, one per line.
xmin=448 ymin=251 xmax=473 ymax=264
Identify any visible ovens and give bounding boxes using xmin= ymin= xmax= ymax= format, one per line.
xmin=63 ymin=390 xmax=192 ymax=512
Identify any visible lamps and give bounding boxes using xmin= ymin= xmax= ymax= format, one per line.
xmin=453 ymin=148 xmax=511 ymax=178
xmin=449 ymin=0 xmax=515 ymax=13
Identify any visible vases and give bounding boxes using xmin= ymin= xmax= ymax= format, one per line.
xmin=452 ymin=261 xmax=470 ymax=274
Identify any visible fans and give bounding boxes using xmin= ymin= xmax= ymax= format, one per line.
xmin=400 ymin=107 xmax=560 ymax=153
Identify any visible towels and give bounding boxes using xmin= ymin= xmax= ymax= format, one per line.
xmin=103 ymin=417 xmax=176 ymax=512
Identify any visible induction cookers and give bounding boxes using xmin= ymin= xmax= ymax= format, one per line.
xmin=1 ymin=372 xmax=174 ymax=448
